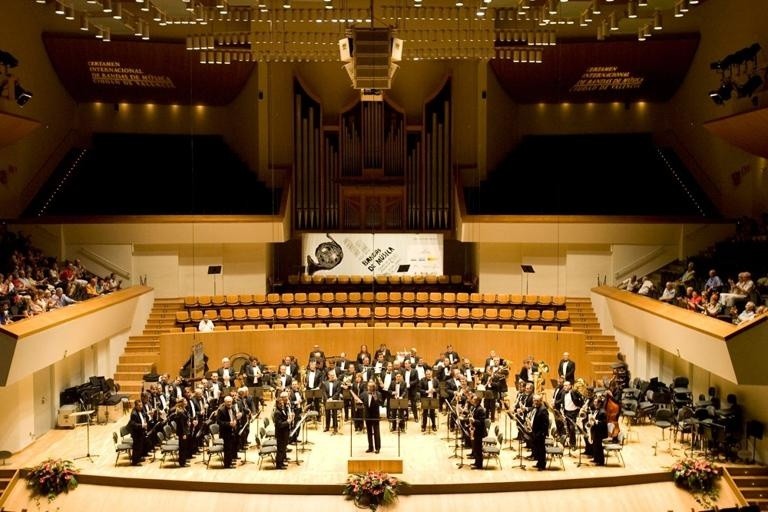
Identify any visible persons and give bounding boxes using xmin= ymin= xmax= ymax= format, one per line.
xmin=304 ymin=344 xmax=438 ymax=433
xmin=0 ymin=223 xmax=123 ymax=325
xmin=433 ymin=345 xmax=508 ymax=469
xmin=127 ymin=353 xmax=307 ymax=471
xmin=511 ymin=356 xmax=550 ymax=470
xmin=356 ymin=381 xmax=383 ymax=454
xmin=199 ymin=314 xmax=215 ymax=331
xmin=616 ymin=261 xmax=768 ymax=325
xmin=552 ymin=351 xmax=630 ymax=467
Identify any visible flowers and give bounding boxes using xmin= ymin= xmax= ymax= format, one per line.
xmin=20 ymin=458 xmax=81 ymax=509
xmin=341 ymin=468 xmax=410 ymax=512
xmin=669 ymin=456 xmax=724 ymax=509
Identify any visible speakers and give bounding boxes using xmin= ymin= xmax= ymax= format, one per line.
xmin=482 ymin=91 xmax=486 ymax=98
xmin=259 ymin=92 xmax=263 ymax=100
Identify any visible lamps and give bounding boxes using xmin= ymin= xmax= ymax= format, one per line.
xmin=706 ymin=43 xmax=766 ymax=105
xmin=19 ymin=0 xmax=699 ymax=67
xmin=11 ymin=78 xmax=34 ymax=109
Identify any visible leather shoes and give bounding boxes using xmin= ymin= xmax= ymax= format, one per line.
xmin=466 ymin=454 xmax=475 ymax=459
xmin=470 ymin=464 xmax=478 ymax=469
xmin=224 ymin=464 xmax=288 ymax=470
xmin=323 ymin=425 xmax=437 ymax=432
xmin=366 ymin=449 xmax=380 ymax=454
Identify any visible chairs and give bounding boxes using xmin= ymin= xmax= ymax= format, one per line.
xmin=170 ymin=293 xmax=575 ymax=334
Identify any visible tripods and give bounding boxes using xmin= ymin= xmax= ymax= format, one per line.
xmin=747 ymin=436 xmax=764 ymax=466
xmin=73 ymin=417 xmax=99 ymax=464
xmin=148 ymin=398 xmax=589 ymax=471
